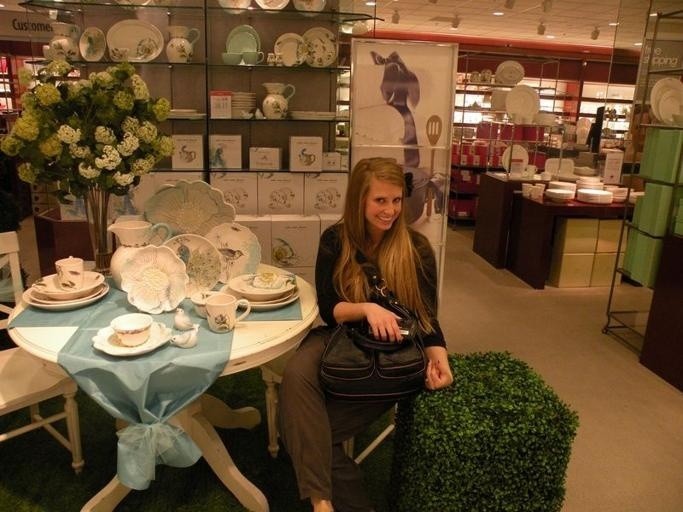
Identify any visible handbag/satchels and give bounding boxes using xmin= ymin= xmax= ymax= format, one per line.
xmin=319 ymin=276 xmax=429 ymax=402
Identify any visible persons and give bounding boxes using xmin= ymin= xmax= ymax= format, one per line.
xmin=279 ymin=157 xmax=454 ymax=512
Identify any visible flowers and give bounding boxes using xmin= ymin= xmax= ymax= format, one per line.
xmin=0 ymin=60 xmax=175 ymax=275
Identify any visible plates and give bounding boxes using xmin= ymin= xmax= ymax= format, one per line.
xmin=549 ymin=181 xmax=577 ymax=192
xmin=220 ymin=284 xmax=301 ymax=312
xmin=301 ymin=27 xmax=339 ymax=67
xmin=650 ymin=78 xmax=683 ymax=124
xmin=28 ymin=285 xmax=104 ymax=304
xmin=274 ymin=33 xmax=307 ymax=68
xmin=92 ymin=321 xmax=172 ymax=357
xmin=163 ymin=234 xmax=222 ymax=298
xmin=293 ymin=0 xmax=326 ymax=17
xmin=226 ymin=24 xmax=261 ymax=53
xmin=228 ymin=274 xmax=297 ymax=301
xmin=143 ymin=178 xmax=236 ymax=242
xmin=218 ymin=0 xmax=252 ymax=14
xmin=223 ymin=286 xmax=295 ymax=305
xmin=545 ymin=189 xmax=575 ymax=203
xmin=289 ymin=110 xmax=335 ymax=121
xmin=576 ymin=180 xmax=604 ymax=190
xmin=496 ymin=61 xmax=525 ymax=84
xmin=31 ymin=271 xmax=105 ymax=299
xmin=580 ymin=177 xmax=600 ymax=183
xmin=657 ymin=89 xmax=683 ymax=125
xmin=170 ymin=109 xmax=197 ymax=115
xmin=577 ymin=189 xmax=613 ymax=204
xmin=79 ymin=27 xmax=106 ymax=62
xmin=228 ymin=32 xmax=257 ymax=65
xmin=22 ymin=282 xmax=109 ymax=312
xmin=204 ymin=223 xmax=261 ymax=285
xmin=107 ymin=19 xmax=164 ymax=63
xmin=255 ymin=0 xmax=290 ymax=14
xmin=505 ymin=85 xmax=540 ymax=120
xmin=502 ymin=145 xmax=528 ymax=172
xmin=119 ymin=245 xmax=189 ymax=315
xmin=171 ymin=114 xmax=206 ymax=119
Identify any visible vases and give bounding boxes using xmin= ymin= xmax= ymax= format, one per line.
xmin=83 ymin=186 xmax=114 ymax=277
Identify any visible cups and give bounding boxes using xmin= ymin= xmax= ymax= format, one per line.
xmin=55 ymin=256 xmax=84 ymax=291
xmin=112 ymin=47 xmax=129 ymax=62
xmin=522 ymin=183 xmax=532 ymax=197
xmin=206 ymin=294 xmax=250 ymax=333
xmin=527 ymin=164 xmax=537 ymax=177
xmin=536 ymin=183 xmax=545 ymax=196
xmin=528 ymin=186 xmax=540 ymax=199
xmin=242 ymin=52 xmax=264 ymax=65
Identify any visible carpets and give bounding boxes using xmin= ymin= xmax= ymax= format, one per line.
xmin=0 ymin=350 xmax=577 ymax=511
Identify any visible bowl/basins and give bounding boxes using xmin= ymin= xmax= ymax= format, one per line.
xmin=613 ymin=196 xmax=626 ymax=203
xmin=111 ymin=313 xmax=153 ymax=346
xmin=191 ymin=292 xmax=224 ymax=320
xmin=222 ymin=53 xmax=242 ymax=64
xmin=231 ymin=92 xmax=257 ymax=120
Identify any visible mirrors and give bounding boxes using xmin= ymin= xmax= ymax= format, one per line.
xmin=596 ymin=1 xmax=650 ymax=178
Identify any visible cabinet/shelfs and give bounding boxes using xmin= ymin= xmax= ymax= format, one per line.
xmin=0 ymin=42 xmax=81 ymax=134
xmin=487 ymin=122 xmax=553 ymax=181
xmin=602 ymin=1 xmax=683 ymax=355
xmin=449 ymin=52 xmax=560 ymax=232
xmin=19 ymin=0 xmax=384 ymax=173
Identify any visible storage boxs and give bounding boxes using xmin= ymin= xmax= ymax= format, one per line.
xmin=319 ymin=216 xmax=342 ymax=238
xmin=232 ymin=215 xmax=271 ymax=266
xmin=270 ymin=216 xmax=321 ymax=267
xmin=551 ymin=215 xmax=598 ymax=253
xmin=595 ymin=219 xmax=628 ymax=253
xmin=589 ymin=252 xmax=624 ymax=288
xmin=151 ymin=172 xmax=205 ymax=191
xmin=257 ymin=172 xmax=304 ymax=215
xmin=548 ymin=253 xmax=593 ymax=287
xmin=289 ymin=136 xmax=322 ymax=171
xmin=322 ymin=151 xmax=340 ymax=170
xmin=249 ymin=147 xmax=281 ymax=170
xmin=172 ymin=133 xmax=204 ymax=169
xmin=304 ymin=173 xmax=348 ymax=216
xmin=209 ymin=134 xmax=241 ymax=169
xmin=208 ymin=173 xmax=257 ymax=215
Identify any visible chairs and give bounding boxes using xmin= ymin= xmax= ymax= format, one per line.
xmin=0 ymin=232 xmax=84 ymax=475
xmin=260 ymin=341 xmax=399 ymax=467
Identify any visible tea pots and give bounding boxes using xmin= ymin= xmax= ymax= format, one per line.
xmin=260 ymin=82 xmax=296 ymax=119
xmin=49 ymin=22 xmax=81 ymax=61
xmin=107 ymin=221 xmax=172 ymax=293
xmin=166 ymin=25 xmax=200 ymax=63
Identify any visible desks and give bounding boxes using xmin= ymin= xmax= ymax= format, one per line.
xmin=506 ymin=195 xmax=638 ymax=291
xmin=472 ymin=172 xmax=578 ymax=270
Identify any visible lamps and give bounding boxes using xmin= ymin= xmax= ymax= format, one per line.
xmin=504 ymin=0 xmax=514 ymax=8
xmin=542 ymin=0 xmax=551 ymax=12
xmin=453 ymin=16 xmax=459 ymax=27
xmin=537 ymin=22 xmax=545 ymax=35
xmin=591 ymin=28 xmax=601 ymax=38
xmin=391 ymin=11 xmax=399 ymax=24
